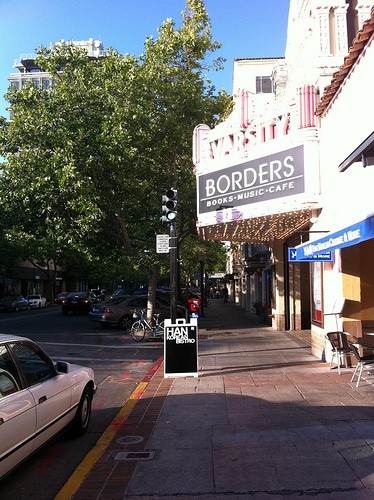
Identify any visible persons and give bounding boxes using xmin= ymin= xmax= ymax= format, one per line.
xmin=191 ymin=283 xmax=228 ymax=303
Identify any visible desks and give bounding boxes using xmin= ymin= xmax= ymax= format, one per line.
xmin=357 ymin=336 xmax=374 ymax=375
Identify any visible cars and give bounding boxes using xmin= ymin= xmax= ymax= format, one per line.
xmin=2 ymin=294 xmax=47 ymax=311
xmin=0 ymin=333 xmax=97 ymax=479
xmin=56 ymin=288 xmax=186 ymax=329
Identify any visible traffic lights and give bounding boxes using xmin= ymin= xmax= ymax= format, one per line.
xmin=161 ymin=190 xmax=179 ymax=223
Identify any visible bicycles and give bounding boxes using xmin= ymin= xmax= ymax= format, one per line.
xmin=131 ymin=308 xmax=164 ymax=342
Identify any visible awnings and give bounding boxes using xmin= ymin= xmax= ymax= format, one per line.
xmin=295 ymin=212 xmax=374 ymax=261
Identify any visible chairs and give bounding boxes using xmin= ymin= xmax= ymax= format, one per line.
xmin=348 ymin=343 xmax=374 ymax=387
xmin=325 ymin=332 xmax=360 ymax=375
